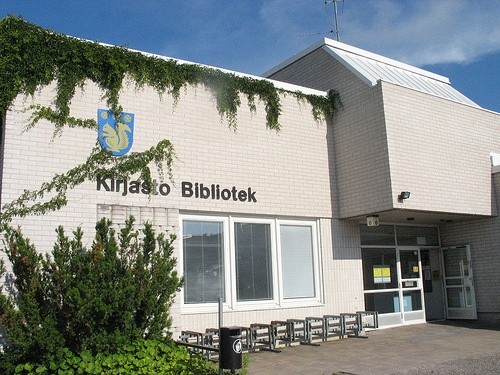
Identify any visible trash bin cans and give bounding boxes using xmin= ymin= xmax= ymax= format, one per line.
xmin=219 ymin=327 xmax=243 ymax=369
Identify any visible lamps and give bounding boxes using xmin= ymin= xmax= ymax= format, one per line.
xmin=398 ymin=192 xmax=410 ymax=200
xmin=366 ymin=215 xmax=379 ymax=227
